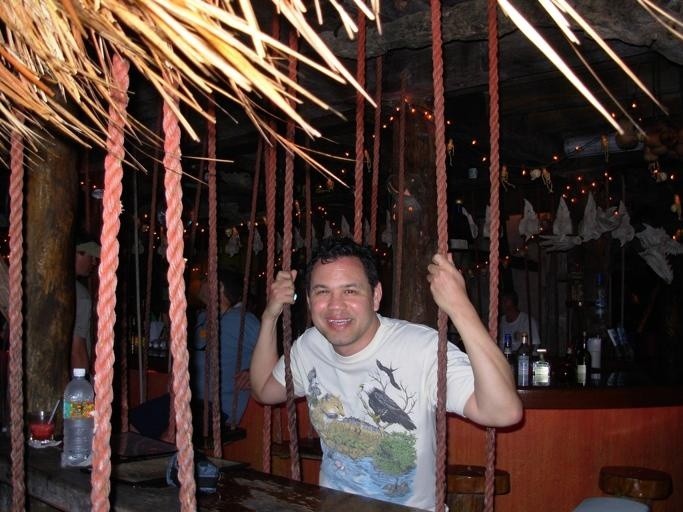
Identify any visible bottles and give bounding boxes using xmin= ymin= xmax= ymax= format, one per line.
xmin=574 ymin=329 xmax=592 ymax=388
xmin=516 ymin=332 xmax=531 ymax=389
xmin=501 ymin=333 xmax=516 ymax=385
xmin=449 ymin=198 xmax=473 ymax=247
xmin=593 ymin=272 xmax=608 ymax=323
xmin=530 ymin=348 xmax=552 ymax=388
xmin=61 ymin=367 xmax=98 ymax=468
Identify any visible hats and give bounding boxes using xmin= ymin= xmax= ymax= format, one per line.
xmin=76 ymin=241 xmax=101 ymax=258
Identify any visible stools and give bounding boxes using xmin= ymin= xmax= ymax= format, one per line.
xmin=598 ymin=464 xmax=674 ymax=512
xmin=446 ymin=464 xmax=511 ymax=510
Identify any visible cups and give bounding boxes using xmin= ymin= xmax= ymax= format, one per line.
xmin=25 ymin=407 xmax=56 ymax=445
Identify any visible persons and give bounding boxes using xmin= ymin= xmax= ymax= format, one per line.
xmin=68 ymin=234 xmax=103 ymax=385
xmin=495 ymin=290 xmax=543 ymax=354
xmin=125 ymin=250 xmax=263 ymax=450
xmin=249 ymin=238 xmax=526 ymax=512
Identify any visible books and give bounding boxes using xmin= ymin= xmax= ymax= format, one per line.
xmin=52 ymin=431 xmax=250 ymax=489
